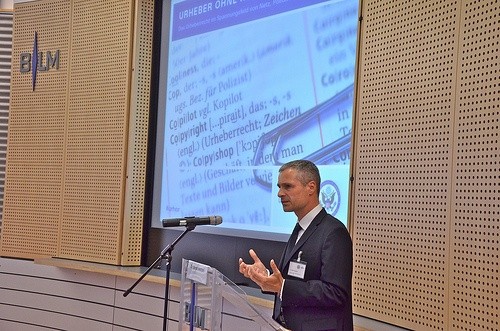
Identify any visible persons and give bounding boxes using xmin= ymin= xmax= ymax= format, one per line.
xmin=239 ymin=159 xmax=353 ymax=331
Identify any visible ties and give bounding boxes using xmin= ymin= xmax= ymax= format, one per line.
xmin=275 ymin=223 xmax=302 ymax=319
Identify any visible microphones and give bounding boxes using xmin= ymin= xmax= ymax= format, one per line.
xmin=162 ymin=216 xmax=222 ymax=227
